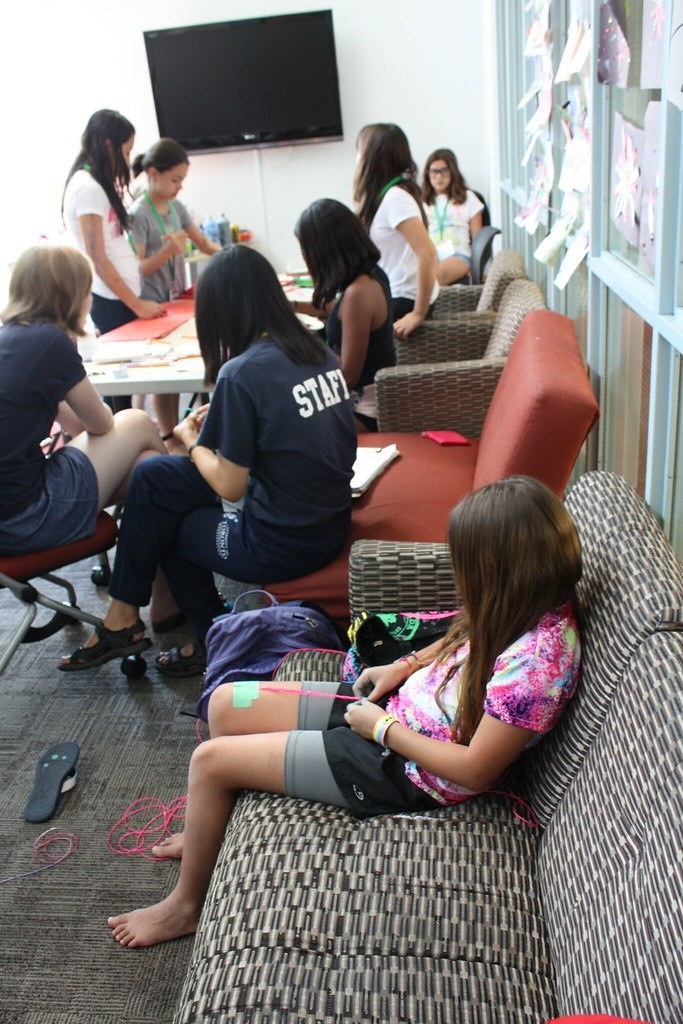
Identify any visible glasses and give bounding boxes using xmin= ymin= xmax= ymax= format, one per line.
xmin=426 ymin=167 xmax=449 ymax=178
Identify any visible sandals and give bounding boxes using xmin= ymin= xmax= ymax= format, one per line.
xmin=153 ymin=634 xmax=206 ymax=677
xmin=56 ymin=618 xmax=152 ymax=672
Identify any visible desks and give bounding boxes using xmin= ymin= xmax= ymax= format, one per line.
xmin=82 ymin=274 xmax=341 ymax=406
xmin=183 ymin=241 xmax=263 ymax=290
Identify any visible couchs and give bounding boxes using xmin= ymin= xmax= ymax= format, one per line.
xmin=173 ymin=470 xmax=682 ymax=1023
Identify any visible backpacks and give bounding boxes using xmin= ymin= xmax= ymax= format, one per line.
xmin=195 ymin=590 xmax=346 ymax=722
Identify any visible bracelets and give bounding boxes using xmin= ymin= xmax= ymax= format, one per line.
xmin=188 ymin=442 xmax=202 ymax=457
xmin=372 ymin=714 xmax=400 ymax=747
xmin=294 ymin=300 xmax=298 ymax=311
xmin=400 ymin=654 xmax=419 ymax=672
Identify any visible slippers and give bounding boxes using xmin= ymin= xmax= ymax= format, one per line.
xmin=23 ymin=742 xmax=81 ymax=823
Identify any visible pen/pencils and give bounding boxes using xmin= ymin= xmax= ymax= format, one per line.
xmin=128 ymin=360 xmax=171 ymax=369
xmin=149 ymin=338 xmax=171 ymax=345
xmin=180 ymin=335 xmax=197 ymax=339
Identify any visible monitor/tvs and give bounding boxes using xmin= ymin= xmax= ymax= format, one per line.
xmin=143 ymin=10 xmax=344 ymax=155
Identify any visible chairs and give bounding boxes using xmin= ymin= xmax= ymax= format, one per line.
xmin=374 ymin=249 xmax=550 ymax=437
xmin=0 ymin=510 xmax=148 ymax=678
xmin=265 ymin=310 xmax=601 ymax=635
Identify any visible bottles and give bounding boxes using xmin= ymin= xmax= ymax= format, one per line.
xmin=203 ymin=215 xmax=218 ymax=245
xmin=218 ymin=214 xmax=230 ymax=246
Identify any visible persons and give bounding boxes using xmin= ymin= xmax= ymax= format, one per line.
xmin=352 ymin=122 xmax=440 ymax=342
xmin=288 ymin=199 xmax=398 ymax=434
xmin=63 ymin=110 xmax=223 ymax=461
xmin=107 ymin=474 xmax=583 ymax=947
xmin=56 ymin=245 xmax=356 ymax=677
xmin=420 ymin=148 xmax=484 ymax=285
xmin=0 ymin=241 xmax=226 ymax=635
